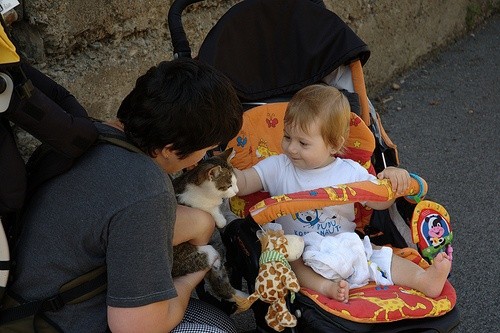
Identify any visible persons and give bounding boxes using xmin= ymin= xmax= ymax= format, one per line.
xmin=5 ymin=59 xmax=239 ymax=333
xmin=231 ymin=85 xmax=451 ymax=304
xmin=0 ymin=5 xmax=124 ymax=251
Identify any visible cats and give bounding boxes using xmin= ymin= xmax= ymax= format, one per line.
xmin=172 ymin=147 xmax=250 ymax=302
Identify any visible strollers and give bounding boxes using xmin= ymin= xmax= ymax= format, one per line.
xmin=166 ymin=0 xmax=458 ymax=333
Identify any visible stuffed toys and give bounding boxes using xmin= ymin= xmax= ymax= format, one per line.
xmin=232 ymin=223 xmax=305 ymax=333
